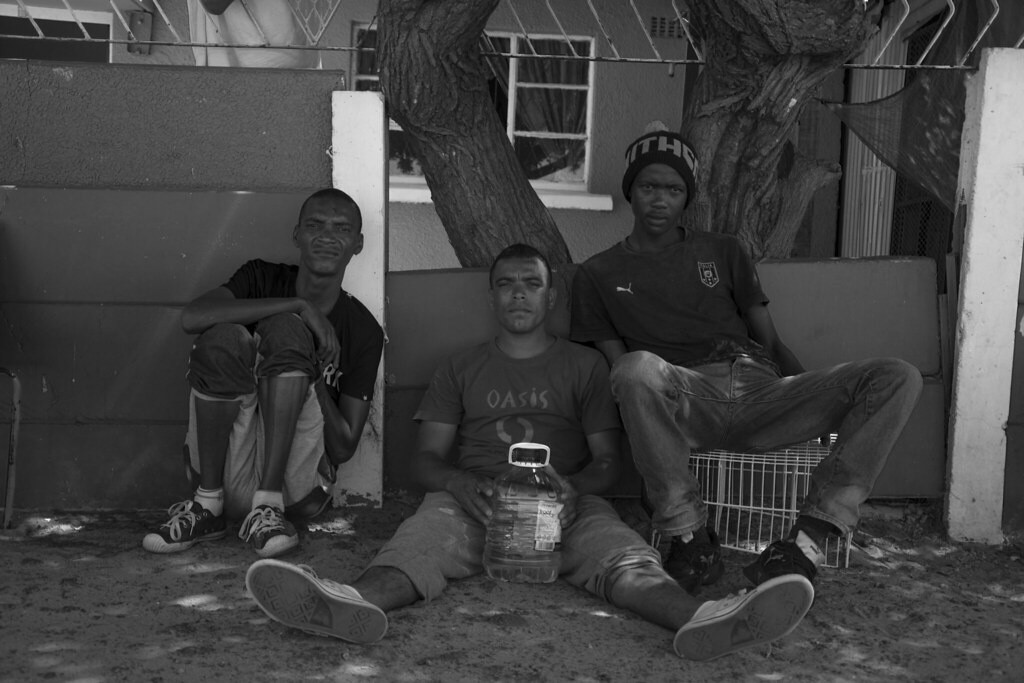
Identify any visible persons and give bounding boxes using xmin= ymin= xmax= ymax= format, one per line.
xmin=142 ymin=189 xmax=383 ymax=560
xmin=568 ymin=120 xmax=924 ymax=585
xmin=245 ymin=243 xmax=814 ymax=663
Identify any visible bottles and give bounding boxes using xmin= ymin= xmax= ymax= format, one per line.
xmin=482 ymin=440 xmax=565 ymax=585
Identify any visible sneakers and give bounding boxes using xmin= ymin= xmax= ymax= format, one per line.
xmin=242 ymin=506 xmax=308 ymax=561
xmin=143 ymin=500 xmax=232 ymax=553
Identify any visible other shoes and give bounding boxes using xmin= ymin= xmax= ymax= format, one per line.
xmin=671 ymin=573 xmax=814 ymax=664
xmin=246 ymin=559 xmax=391 ymax=645
xmin=745 ymin=541 xmax=821 ymax=596
xmin=669 ymin=528 xmax=728 ymax=595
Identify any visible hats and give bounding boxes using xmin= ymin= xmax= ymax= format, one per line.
xmin=618 ymin=120 xmax=700 ymax=211
